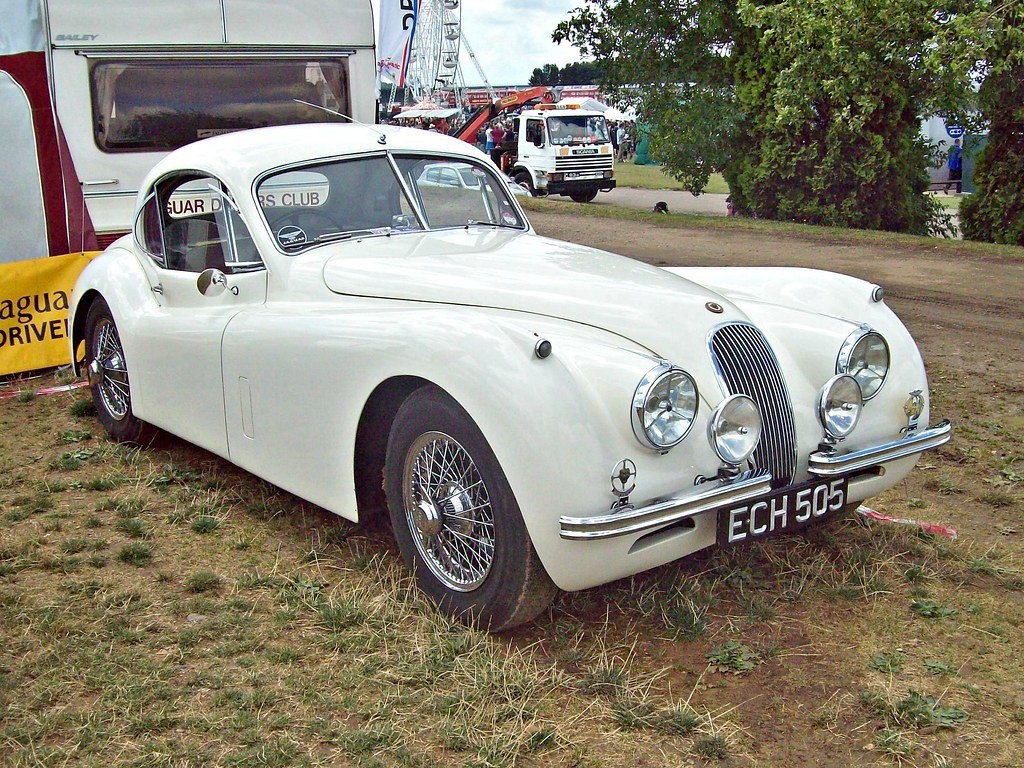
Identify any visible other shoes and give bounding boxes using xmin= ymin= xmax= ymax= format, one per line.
xmin=943 ymin=186 xmax=948 ymax=195
xmin=618 ymin=158 xmax=622 ymax=162
xmin=623 ymin=159 xmax=629 ymax=163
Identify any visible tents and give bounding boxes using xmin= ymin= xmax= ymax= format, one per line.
xmin=557 ymin=97 xmax=641 ymax=120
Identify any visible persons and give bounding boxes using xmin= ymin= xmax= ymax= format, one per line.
xmin=382 ymin=106 xmax=515 ymax=154
xmin=608 ymin=120 xmax=637 ymax=162
xmin=943 ymin=139 xmax=963 ymax=194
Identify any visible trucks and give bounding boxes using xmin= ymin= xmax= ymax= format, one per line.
xmin=490 ymin=104 xmax=616 ymax=203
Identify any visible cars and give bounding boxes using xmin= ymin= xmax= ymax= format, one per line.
xmin=416 ymin=162 xmax=532 ymax=197
xmin=68 ymin=123 xmax=951 ymax=633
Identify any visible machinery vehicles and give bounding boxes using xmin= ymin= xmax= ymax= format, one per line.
xmin=438 ymin=85 xmax=556 ymax=164
